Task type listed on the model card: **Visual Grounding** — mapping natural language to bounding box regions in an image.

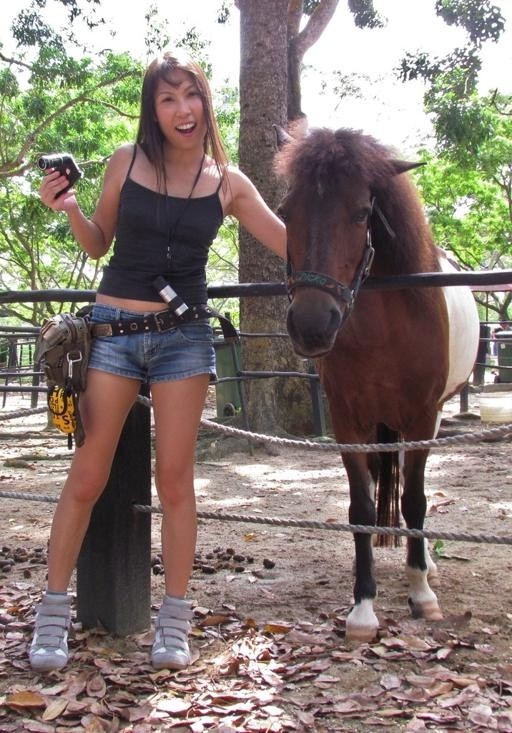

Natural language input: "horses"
[273,122,480,644]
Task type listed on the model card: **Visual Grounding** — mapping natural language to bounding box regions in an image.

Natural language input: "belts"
[93,304,240,347]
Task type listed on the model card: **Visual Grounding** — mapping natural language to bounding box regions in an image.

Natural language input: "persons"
[28,50,289,672]
[500,319,512,331]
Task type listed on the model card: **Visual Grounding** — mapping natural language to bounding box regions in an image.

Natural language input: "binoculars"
[149,274,190,318]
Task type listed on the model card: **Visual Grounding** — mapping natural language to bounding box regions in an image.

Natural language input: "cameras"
[38,150,84,198]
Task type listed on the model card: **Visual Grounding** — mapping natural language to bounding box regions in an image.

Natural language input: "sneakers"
[27,594,69,676]
[150,594,195,674]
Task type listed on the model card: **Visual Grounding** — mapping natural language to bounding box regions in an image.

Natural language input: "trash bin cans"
[495,330,512,383]
[213,335,242,417]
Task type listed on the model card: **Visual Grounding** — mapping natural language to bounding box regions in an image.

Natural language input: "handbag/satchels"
[30,309,92,449]
